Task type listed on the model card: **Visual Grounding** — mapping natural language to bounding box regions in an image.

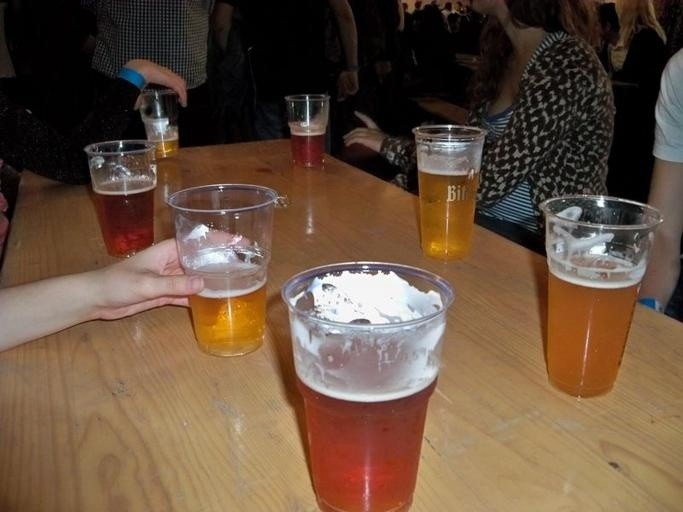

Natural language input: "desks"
[1,135,680,512]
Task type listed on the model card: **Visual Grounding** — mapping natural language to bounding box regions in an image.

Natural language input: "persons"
[630,46,683,311]
[0,228,268,357]
[0,1,682,264]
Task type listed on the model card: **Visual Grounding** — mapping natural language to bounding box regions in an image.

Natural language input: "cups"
[282,92,333,170]
[136,89,182,160]
[82,138,157,257]
[164,181,277,358]
[536,192,664,400]
[281,260,453,512]
[412,124,490,259]
